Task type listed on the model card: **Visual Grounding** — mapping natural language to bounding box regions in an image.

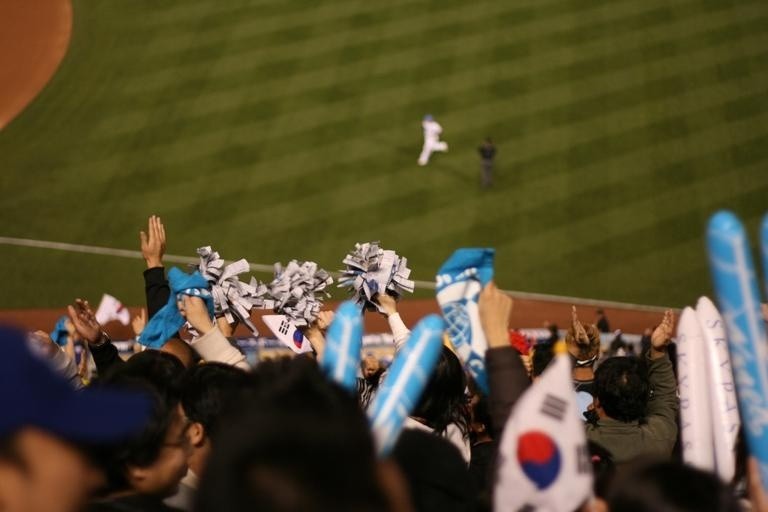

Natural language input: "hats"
[566,322,600,360]
[0,324,151,448]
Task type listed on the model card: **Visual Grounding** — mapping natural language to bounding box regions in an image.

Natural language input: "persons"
[417,116,448,166]
[478,135,498,185]
